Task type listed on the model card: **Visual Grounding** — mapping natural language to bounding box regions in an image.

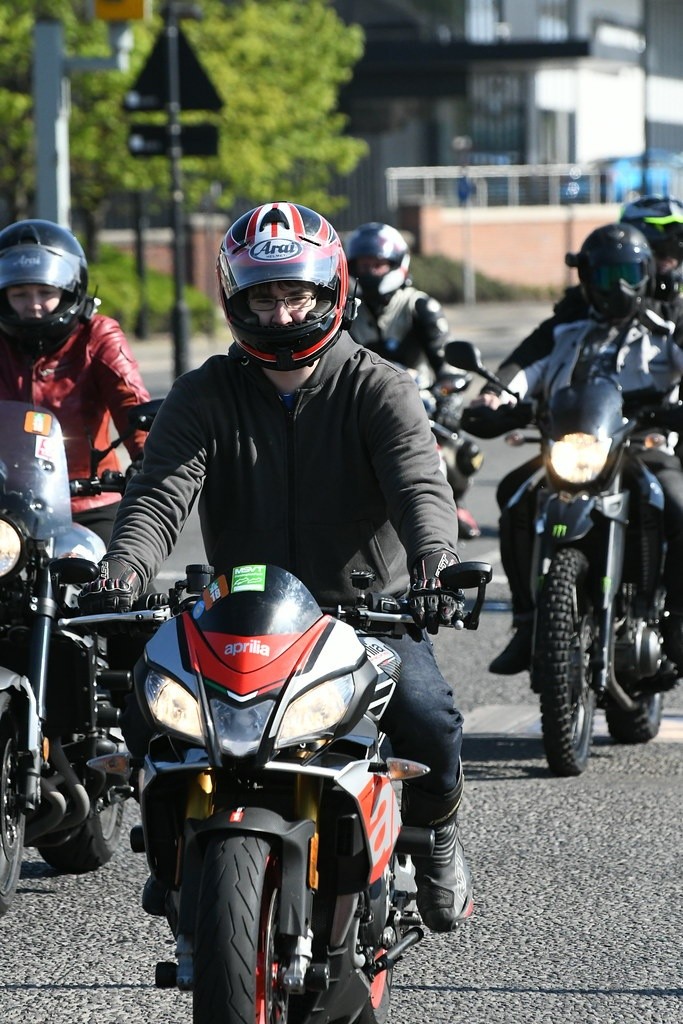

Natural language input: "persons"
[0,220,162,806]
[81,185,475,987]
[461,195,683,676]
[343,220,485,541]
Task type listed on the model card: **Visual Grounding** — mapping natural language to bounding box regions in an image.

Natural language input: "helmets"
[215,202,350,369]
[621,196,683,295]
[578,223,655,321]
[344,222,412,298]
[0,217,88,348]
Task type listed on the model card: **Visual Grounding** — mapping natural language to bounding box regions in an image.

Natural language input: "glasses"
[246,294,319,312]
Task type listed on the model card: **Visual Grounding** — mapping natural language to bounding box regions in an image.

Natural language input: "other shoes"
[416,828,474,932]
[489,633,529,676]
[139,877,164,916]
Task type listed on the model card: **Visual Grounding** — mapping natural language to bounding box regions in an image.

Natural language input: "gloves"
[76,559,148,635]
[404,549,463,644]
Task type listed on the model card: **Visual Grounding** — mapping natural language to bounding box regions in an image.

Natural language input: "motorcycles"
[0,276,681,1024]
